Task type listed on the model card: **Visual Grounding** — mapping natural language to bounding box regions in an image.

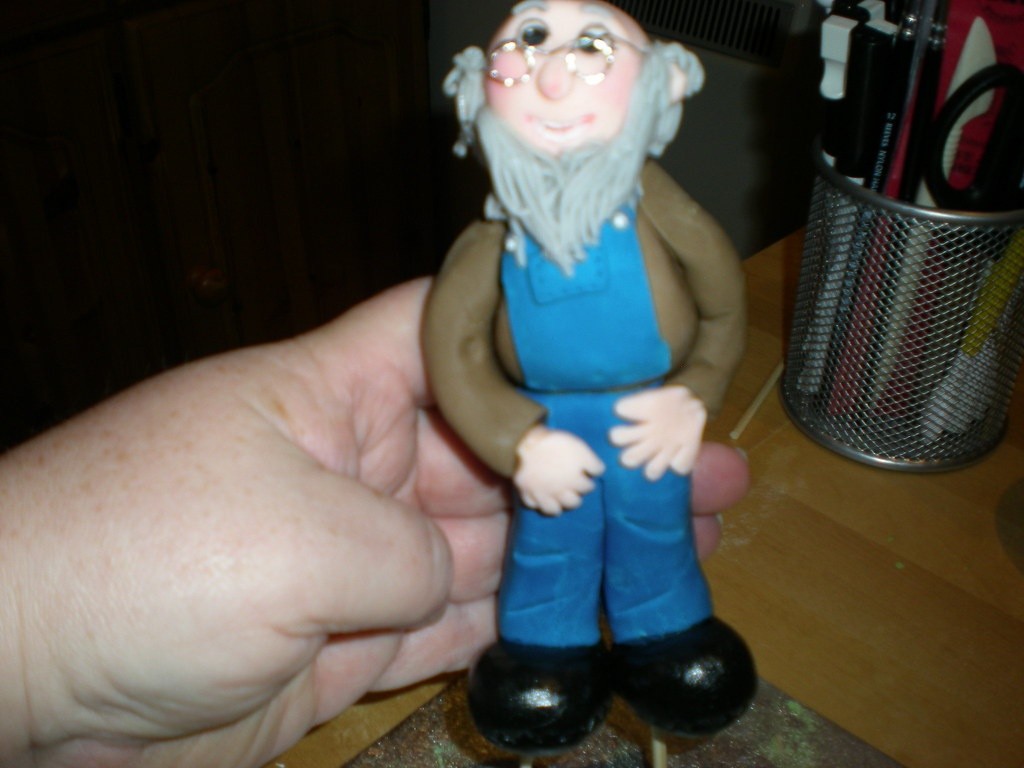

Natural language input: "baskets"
[782,141,1023,471]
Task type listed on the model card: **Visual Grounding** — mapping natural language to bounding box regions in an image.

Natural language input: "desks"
[245,227,1024,768]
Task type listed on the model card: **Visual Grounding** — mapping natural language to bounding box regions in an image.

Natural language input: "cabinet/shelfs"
[0,0,445,455]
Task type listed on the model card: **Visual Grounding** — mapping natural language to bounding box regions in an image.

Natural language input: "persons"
[421,0,759,754]
[0,270,750,767]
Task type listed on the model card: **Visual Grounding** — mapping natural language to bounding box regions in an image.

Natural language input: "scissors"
[909,67,1024,414]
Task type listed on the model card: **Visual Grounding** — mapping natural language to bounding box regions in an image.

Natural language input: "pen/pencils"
[799,0,944,414]
[919,224,1024,441]
